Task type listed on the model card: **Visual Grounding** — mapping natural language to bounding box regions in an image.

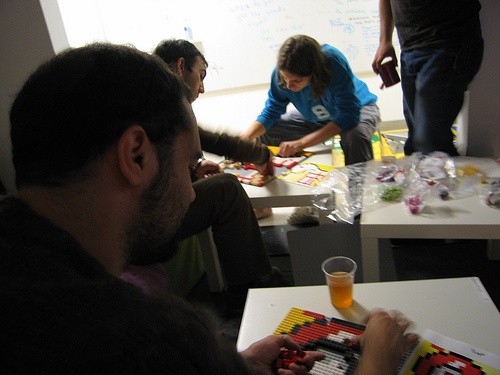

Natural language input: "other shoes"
[254,206,272,221]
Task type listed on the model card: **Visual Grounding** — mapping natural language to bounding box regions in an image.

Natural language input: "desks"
[217,145,337,224]
[359,158,500,283]
[236,276,500,375]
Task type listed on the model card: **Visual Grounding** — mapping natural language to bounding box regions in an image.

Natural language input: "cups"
[321,256,358,311]
[379,60,400,87]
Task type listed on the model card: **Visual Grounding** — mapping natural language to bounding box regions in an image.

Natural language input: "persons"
[151,39,280,293]
[372,0,484,248]
[237,35,382,224]
[0,44,418,375]
